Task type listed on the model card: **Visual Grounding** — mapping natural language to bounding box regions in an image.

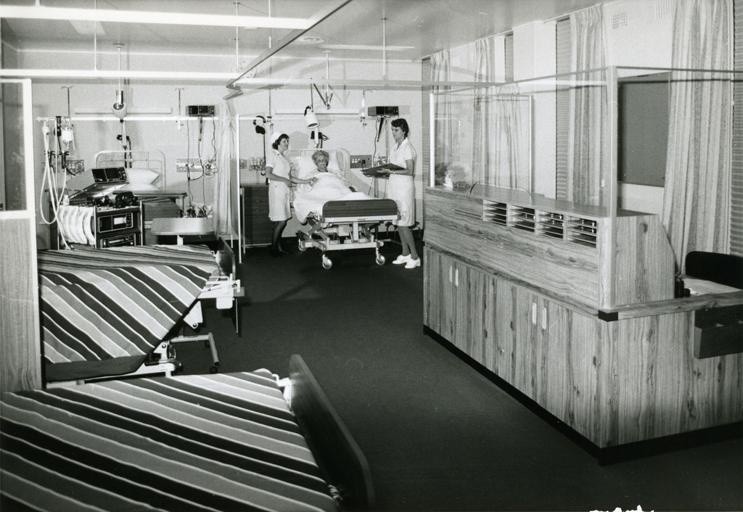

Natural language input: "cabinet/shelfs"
[422,245,608,449]
[238,183,278,248]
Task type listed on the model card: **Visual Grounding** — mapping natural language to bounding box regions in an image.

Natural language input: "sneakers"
[389,252,421,271]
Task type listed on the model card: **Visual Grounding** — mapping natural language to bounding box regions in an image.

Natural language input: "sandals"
[269,243,289,258]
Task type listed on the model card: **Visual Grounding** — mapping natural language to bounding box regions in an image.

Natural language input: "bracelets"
[391,169,395,175]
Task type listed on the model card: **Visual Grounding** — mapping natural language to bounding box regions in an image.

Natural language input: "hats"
[270,131,282,145]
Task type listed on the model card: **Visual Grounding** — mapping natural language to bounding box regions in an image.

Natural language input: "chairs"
[684,251,742,325]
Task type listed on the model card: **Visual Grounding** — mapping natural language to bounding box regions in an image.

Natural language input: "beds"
[285,147,400,271]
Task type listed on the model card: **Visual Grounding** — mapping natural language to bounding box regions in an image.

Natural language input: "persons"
[377,118,421,269]
[303,150,355,191]
[263,128,318,259]
